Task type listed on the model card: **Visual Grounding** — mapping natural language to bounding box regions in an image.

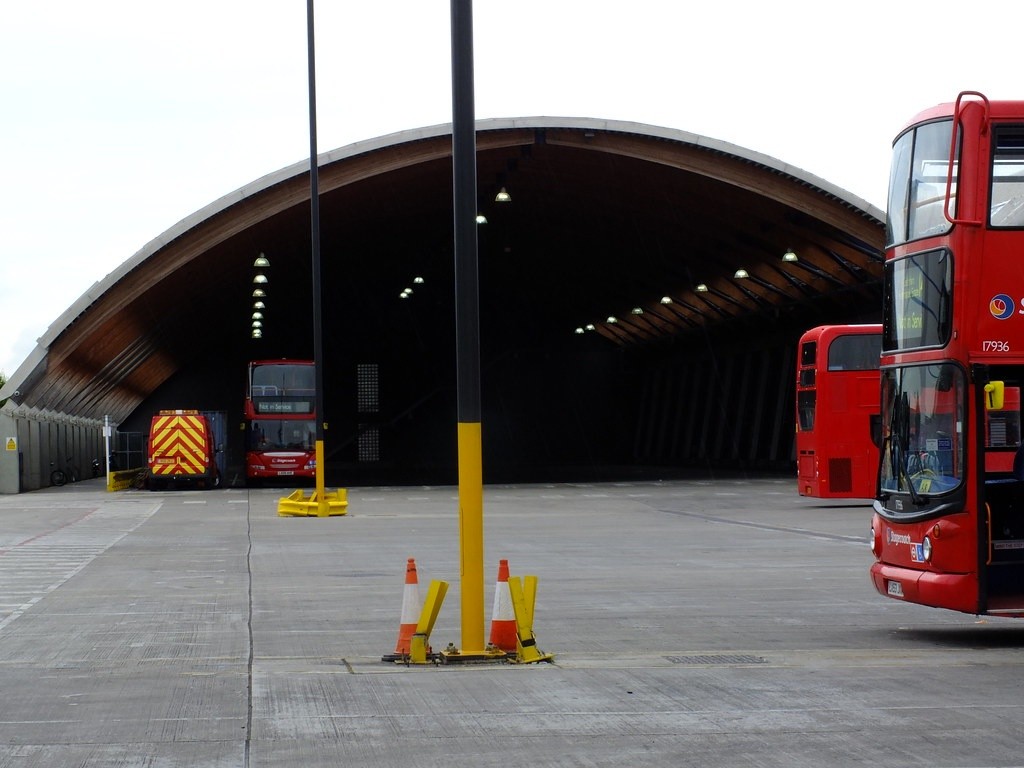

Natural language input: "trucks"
[146,410,228,487]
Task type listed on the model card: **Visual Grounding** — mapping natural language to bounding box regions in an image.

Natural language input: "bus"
[795,324,884,499]
[243,359,316,487]
[870,91,1024,620]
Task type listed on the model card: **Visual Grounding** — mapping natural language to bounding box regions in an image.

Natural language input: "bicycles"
[50,457,78,487]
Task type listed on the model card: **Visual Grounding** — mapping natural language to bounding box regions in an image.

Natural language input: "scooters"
[90,458,102,479]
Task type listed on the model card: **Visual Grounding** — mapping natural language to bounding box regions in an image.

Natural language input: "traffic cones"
[382,557,438,662]
[486,559,520,662]
